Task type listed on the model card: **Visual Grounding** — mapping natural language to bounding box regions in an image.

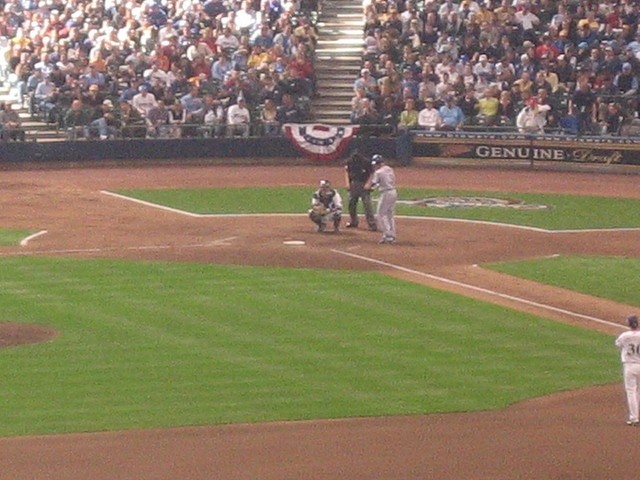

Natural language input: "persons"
[364,154,397,244]
[615,316,640,426]
[308,180,343,236]
[344,147,377,230]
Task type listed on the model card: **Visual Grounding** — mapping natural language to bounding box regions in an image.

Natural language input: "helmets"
[371,154,383,166]
